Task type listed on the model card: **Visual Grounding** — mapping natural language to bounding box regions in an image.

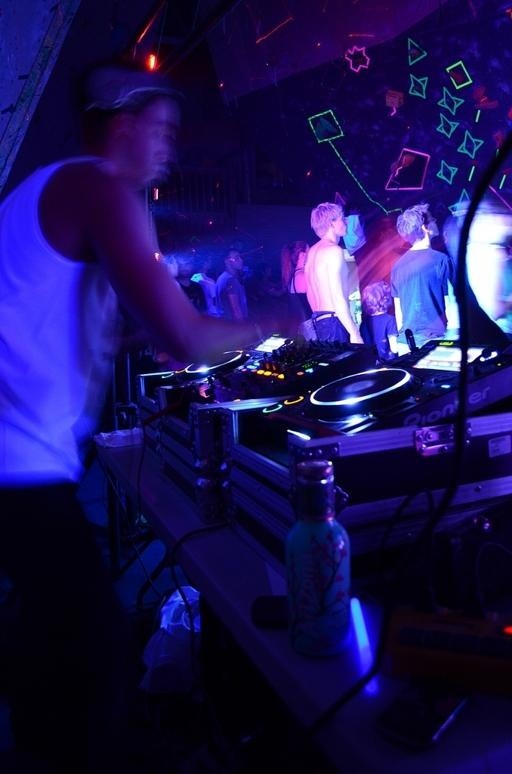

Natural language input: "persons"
[304,203,365,345]
[0,65,312,774]
[487,233,512,334]
[363,284,400,361]
[166,242,315,342]
[390,204,451,354]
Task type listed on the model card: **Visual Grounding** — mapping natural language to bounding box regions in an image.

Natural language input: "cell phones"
[372,679,474,752]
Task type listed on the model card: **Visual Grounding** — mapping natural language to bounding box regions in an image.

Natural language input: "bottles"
[286,460,349,656]
